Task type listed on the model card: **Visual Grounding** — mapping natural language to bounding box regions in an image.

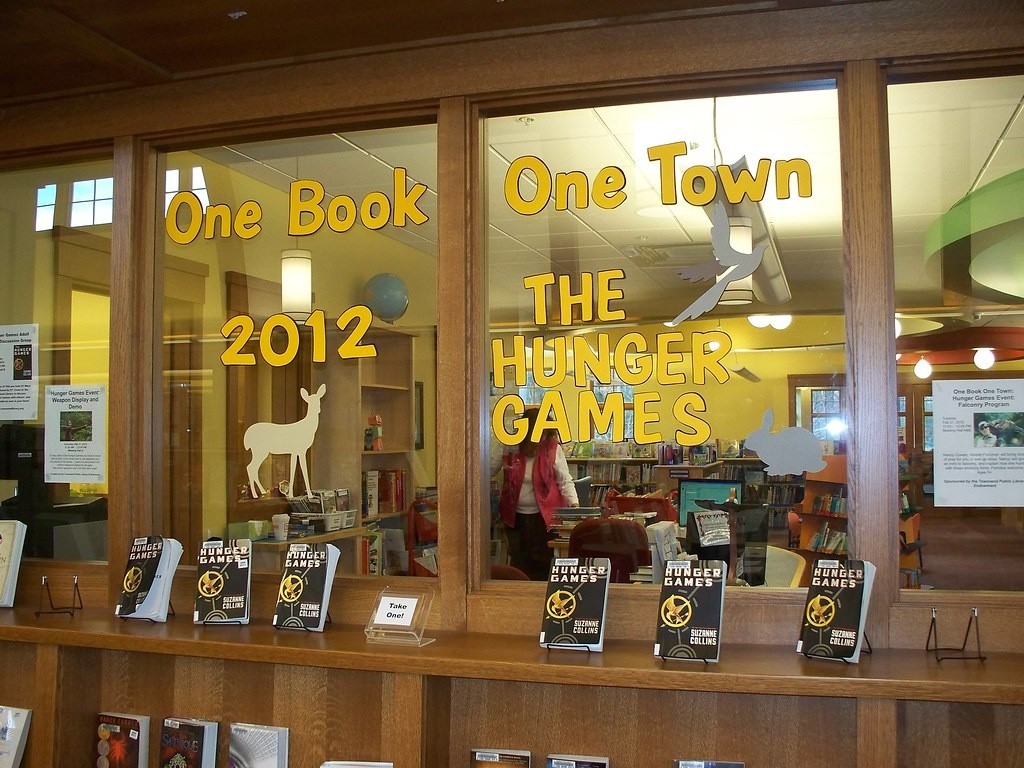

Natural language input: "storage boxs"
[302,509,357,532]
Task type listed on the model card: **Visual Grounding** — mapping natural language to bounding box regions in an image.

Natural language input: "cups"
[272,514,290,541]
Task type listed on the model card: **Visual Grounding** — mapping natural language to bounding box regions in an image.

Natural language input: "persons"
[976,421,996,448]
[490,408,579,581]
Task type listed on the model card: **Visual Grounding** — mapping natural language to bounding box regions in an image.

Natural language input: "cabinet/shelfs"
[310,316,415,527]
[566,456,849,589]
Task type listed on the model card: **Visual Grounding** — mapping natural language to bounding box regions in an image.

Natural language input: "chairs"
[566,519,652,585]
[752,546,806,589]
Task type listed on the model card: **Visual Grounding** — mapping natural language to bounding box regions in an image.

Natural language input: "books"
[0,706,33,768]
[470,749,531,768]
[549,440,804,581]
[546,754,610,768]
[228,723,289,768]
[539,557,611,652]
[0,520,27,608]
[268,468,407,575]
[673,759,745,768]
[796,559,877,664]
[115,536,184,622]
[193,539,252,625]
[272,543,340,632]
[808,492,909,551]
[159,717,218,768]
[320,760,393,768]
[94,712,150,768]
[653,560,727,663]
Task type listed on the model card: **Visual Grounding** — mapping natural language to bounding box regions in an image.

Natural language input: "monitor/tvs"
[678,477,744,527]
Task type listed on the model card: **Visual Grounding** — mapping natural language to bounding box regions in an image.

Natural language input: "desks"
[0,603,1024,768]
[251,526,367,577]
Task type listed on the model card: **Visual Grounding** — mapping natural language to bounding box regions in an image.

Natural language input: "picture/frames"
[415,381,424,450]
